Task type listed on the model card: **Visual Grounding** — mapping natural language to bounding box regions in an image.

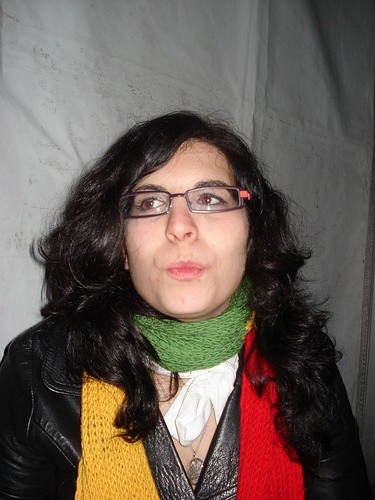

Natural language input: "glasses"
[115,187,250,219]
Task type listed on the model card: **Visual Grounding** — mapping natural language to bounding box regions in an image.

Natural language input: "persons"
[0,111,375,500]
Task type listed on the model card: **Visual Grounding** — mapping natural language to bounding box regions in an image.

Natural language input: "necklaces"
[155,375,211,485]
